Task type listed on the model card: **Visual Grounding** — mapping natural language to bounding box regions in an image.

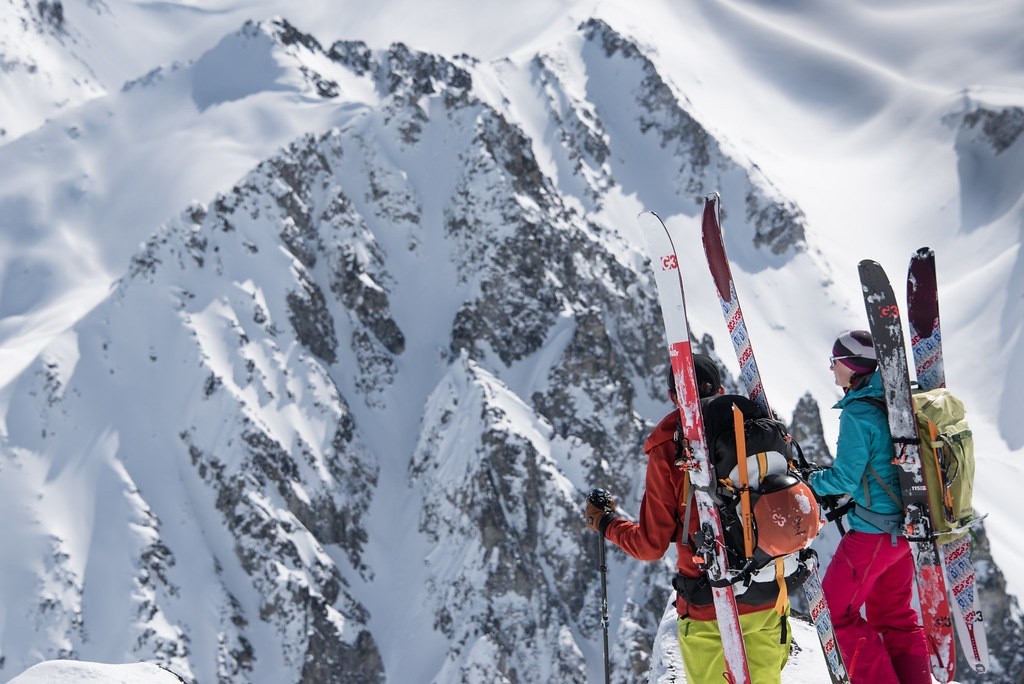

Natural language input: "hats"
[833,330,877,374]
[667,353,722,399]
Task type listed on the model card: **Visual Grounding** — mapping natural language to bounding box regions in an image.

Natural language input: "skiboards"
[634,189,851,683]
[857,245,989,683]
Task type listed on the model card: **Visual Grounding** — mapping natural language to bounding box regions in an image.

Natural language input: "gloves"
[585,493,616,537]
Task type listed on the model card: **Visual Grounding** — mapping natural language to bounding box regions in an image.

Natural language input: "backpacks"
[855,388,977,546]
[673,391,823,606]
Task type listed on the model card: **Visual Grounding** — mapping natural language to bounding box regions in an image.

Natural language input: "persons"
[584,352,793,684]
[789,331,933,684]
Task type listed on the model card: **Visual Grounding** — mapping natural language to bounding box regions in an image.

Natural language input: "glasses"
[829,354,863,366]
